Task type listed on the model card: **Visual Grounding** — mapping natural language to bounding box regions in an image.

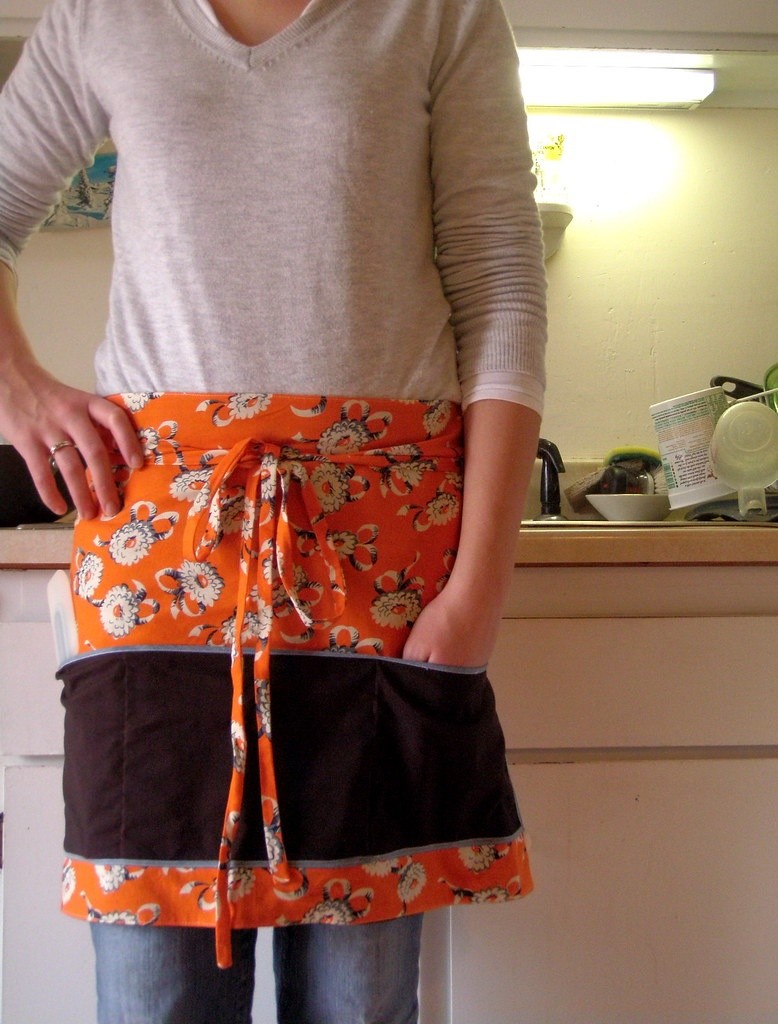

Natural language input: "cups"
[649,386,737,510]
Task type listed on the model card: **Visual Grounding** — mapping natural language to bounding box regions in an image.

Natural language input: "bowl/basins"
[585,494,672,521]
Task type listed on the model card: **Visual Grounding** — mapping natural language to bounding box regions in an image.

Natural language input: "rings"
[50,441,72,453]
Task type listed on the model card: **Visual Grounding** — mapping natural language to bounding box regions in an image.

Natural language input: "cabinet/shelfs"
[0,529,778,1024]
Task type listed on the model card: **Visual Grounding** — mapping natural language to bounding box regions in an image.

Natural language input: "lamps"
[518,62,714,112]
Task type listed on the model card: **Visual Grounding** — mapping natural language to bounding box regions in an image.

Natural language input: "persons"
[0,0,547,1024]
